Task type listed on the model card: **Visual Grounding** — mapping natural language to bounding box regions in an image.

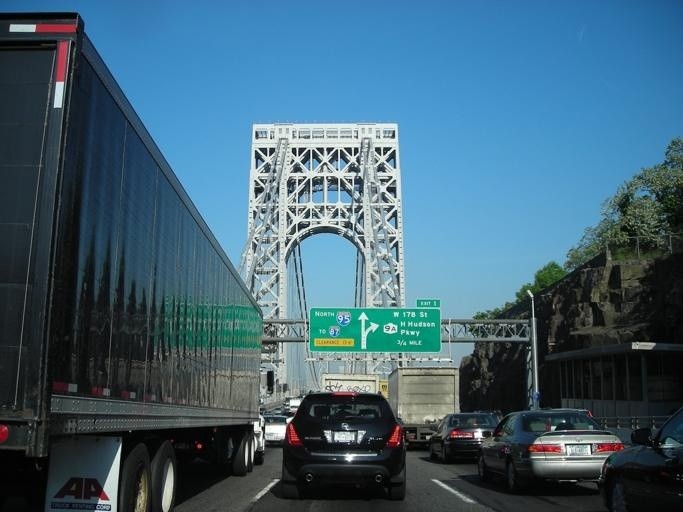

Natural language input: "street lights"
[526,287,540,405]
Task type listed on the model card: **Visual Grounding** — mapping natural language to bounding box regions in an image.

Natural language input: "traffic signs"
[309,307,442,353]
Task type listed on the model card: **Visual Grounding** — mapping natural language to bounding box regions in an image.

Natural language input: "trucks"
[319,372,380,395]
[0,7,275,512]
[387,365,463,451]
